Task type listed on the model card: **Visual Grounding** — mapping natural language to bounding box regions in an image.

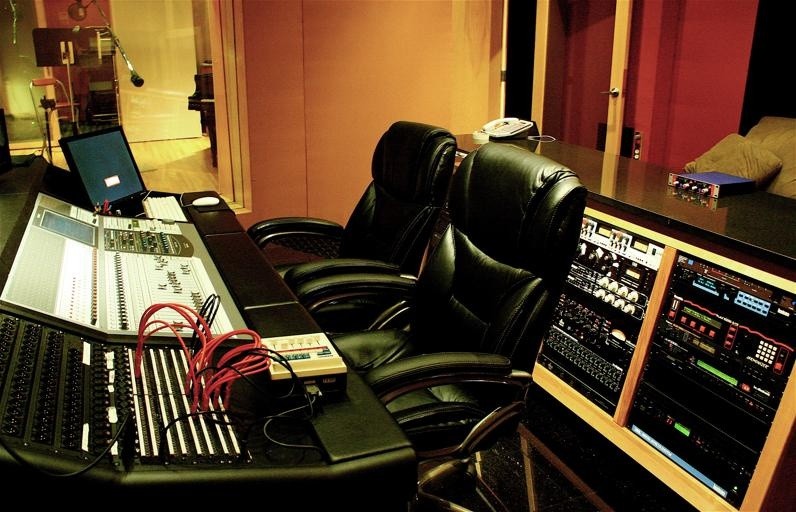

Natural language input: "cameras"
[41,99,55,110]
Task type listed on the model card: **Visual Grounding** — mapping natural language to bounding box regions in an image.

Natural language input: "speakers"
[0,109,13,176]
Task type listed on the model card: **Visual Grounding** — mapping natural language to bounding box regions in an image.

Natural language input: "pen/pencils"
[94,199,112,216]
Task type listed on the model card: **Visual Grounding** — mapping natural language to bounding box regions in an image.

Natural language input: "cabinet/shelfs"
[79,30,122,133]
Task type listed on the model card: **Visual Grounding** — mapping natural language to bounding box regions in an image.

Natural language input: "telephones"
[480,118,540,140]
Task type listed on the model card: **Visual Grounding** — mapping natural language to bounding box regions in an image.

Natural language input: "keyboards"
[141,196,189,222]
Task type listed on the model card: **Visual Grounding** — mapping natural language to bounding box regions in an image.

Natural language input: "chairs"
[28,77,79,166]
[294,142,590,512]
[245,119,459,334]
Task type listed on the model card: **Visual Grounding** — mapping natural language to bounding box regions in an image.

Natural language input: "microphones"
[76,0,85,18]
[23,154,35,167]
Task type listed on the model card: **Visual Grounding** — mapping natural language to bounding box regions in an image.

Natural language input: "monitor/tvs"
[59,125,147,215]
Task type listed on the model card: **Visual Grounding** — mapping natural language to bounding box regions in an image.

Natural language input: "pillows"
[687,132,782,187]
[733,114,796,197]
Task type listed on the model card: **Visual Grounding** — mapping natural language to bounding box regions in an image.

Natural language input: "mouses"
[192,196,220,207]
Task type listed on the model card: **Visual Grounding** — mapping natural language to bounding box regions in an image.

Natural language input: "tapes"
[473,133,489,140]
[472,140,489,144]
